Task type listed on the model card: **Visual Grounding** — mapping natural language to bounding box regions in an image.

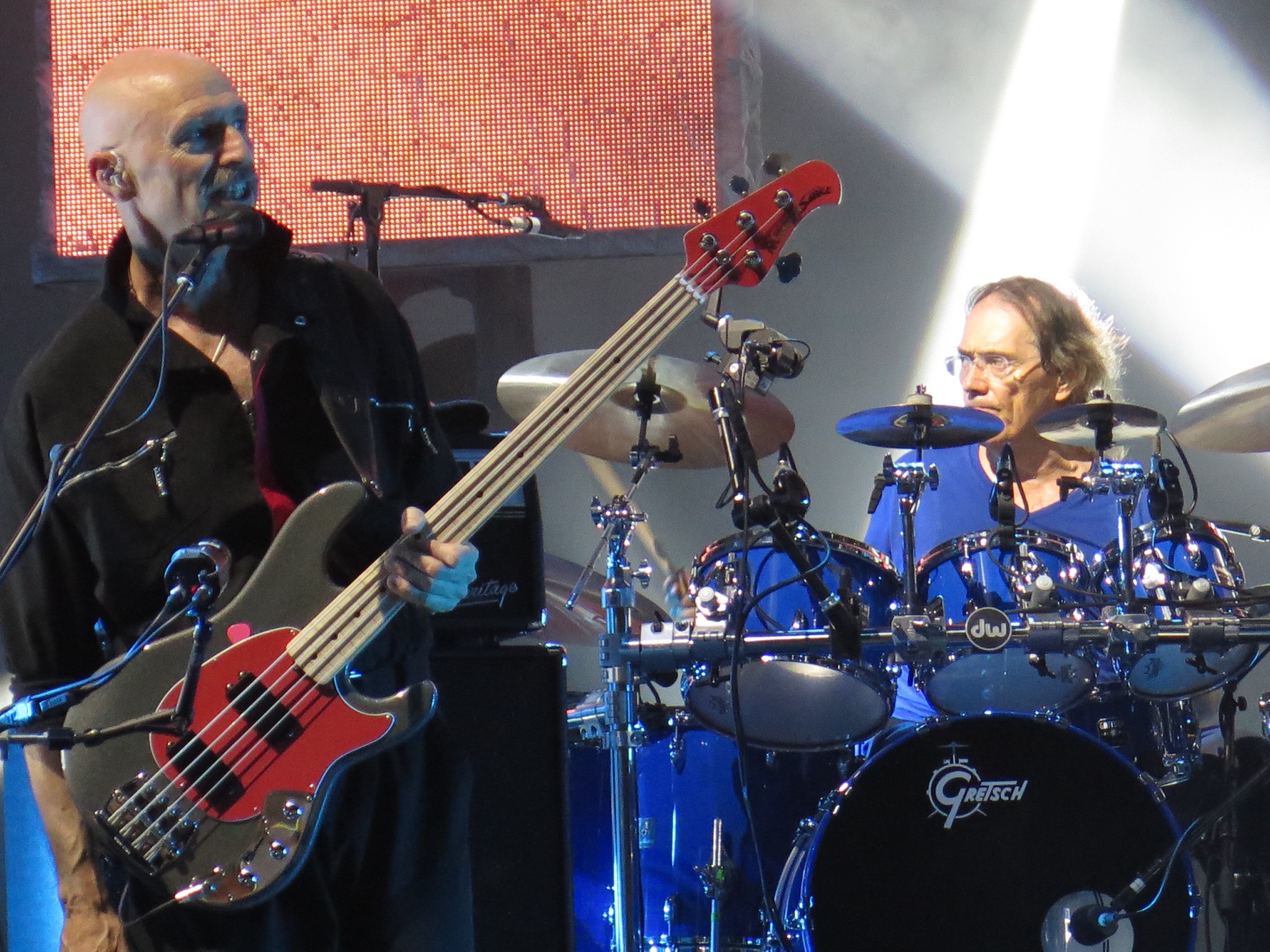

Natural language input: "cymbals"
[1033,399,1167,447]
[496,349,795,470]
[835,405,1005,449]
[1171,362,1270,453]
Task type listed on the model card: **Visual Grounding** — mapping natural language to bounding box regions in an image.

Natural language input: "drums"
[570,729,749,952]
[915,528,1099,716]
[1091,516,1259,702]
[680,528,901,753]
[766,710,1202,952]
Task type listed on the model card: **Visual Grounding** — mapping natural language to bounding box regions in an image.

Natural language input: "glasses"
[945,353,1044,378]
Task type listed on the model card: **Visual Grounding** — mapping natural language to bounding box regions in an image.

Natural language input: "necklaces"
[123,264,260,370]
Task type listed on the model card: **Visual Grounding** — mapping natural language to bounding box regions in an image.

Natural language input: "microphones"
[169,205,265,251]
[498,214,585,240]
[744,326,805,384]
[1145,434,1171,522]
[770,447,797,555]
[988,445,1016,523]
[163,540,231,618]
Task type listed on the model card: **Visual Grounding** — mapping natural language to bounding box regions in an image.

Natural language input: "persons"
[1,44,478,950]
[731,278,1177,924]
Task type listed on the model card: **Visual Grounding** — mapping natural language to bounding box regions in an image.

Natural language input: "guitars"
[60,153,842,910]
